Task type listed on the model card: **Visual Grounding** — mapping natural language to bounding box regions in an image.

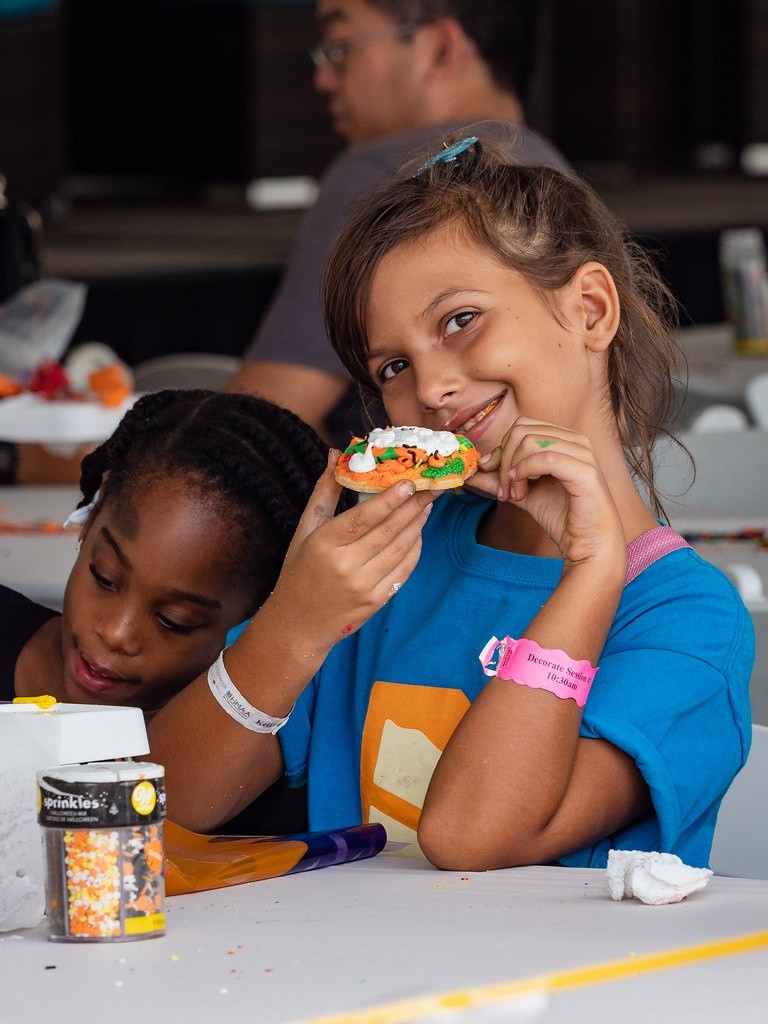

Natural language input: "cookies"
[334,433,481,493]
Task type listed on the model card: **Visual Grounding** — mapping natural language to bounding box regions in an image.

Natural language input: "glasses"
[392,136,483,185]
[313,24,456,73]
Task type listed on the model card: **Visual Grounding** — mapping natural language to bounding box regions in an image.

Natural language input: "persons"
[230,0,590,425]
[149,134,756,875]
[0,390,360,735]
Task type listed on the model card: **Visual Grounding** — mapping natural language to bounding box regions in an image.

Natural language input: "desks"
[46,175,768,283]
[0,856,768,1024]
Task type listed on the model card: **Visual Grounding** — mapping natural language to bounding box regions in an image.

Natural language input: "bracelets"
[479,631,601,707]
[207,644,297,733]
